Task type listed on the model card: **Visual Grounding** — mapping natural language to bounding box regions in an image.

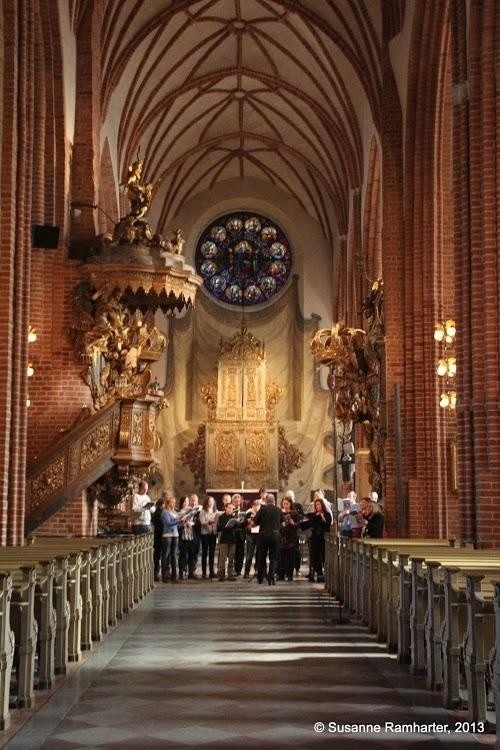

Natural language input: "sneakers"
[154,570,324,585]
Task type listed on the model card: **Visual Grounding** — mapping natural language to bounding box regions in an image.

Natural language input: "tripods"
[248,534,272,583]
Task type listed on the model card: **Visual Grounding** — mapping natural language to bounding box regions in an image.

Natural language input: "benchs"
[0,525,156,734]
[322,527,500,736]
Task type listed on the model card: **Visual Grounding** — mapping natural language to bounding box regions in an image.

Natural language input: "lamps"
[436,356,457,379]
[439,388,457,411]
[433,318,458,347]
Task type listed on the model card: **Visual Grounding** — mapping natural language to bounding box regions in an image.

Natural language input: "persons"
[126,159,148,229]
[132,478,385,585]
